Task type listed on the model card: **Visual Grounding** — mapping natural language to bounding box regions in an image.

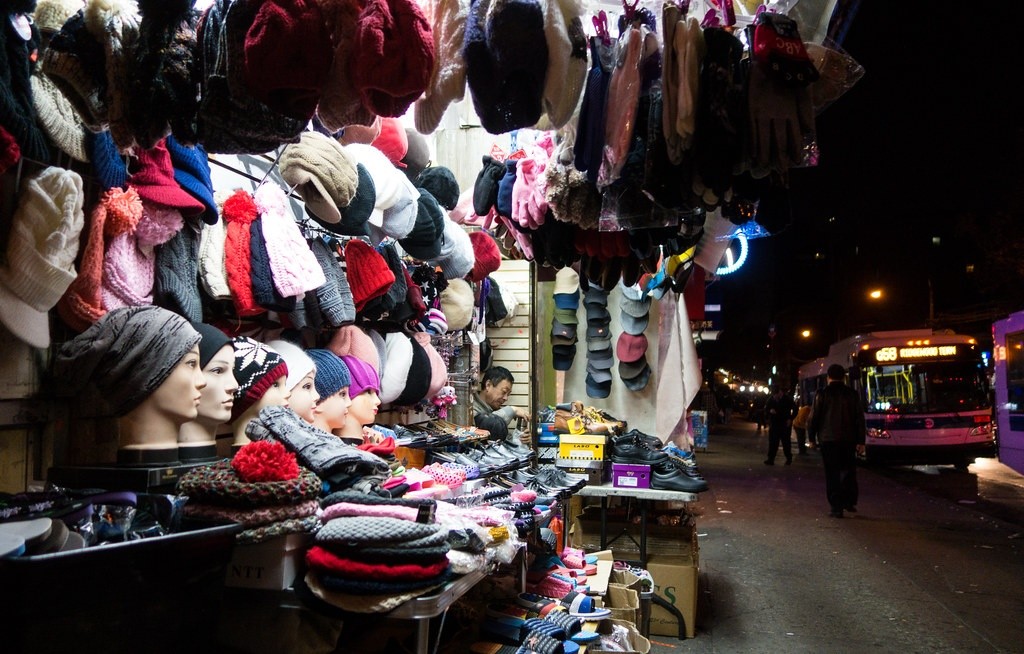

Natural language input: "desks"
[577,482,697,641]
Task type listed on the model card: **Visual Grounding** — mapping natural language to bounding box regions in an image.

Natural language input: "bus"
[797,328,1000,472]
[991,311,1024,475]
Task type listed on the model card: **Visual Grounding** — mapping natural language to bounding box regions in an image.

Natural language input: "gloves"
[470,5,864,293]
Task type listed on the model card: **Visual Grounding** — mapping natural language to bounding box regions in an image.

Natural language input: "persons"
[807,364,866,518]
[266,339,320,422]
[178,322,238,446]
[473,366,531,444]
[230,335,291,446]
[56,306,208,448]
[755,392,766,431]
[333,354,382,439]
[763,386,799,465]
[794,405,811,456]
[303,349,352,434]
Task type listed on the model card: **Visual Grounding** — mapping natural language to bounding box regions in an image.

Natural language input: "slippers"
[492,543,654,654]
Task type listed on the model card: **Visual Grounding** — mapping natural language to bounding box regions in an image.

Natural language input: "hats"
[550,262,693,398]
[175,408,538,613]
[0,0,570,426]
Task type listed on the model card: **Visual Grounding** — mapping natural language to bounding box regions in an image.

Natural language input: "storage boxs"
[569,506,699,654]
[389,445,423,470]
[537,419,611,485]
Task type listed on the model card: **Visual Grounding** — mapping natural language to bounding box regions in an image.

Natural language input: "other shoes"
[763,459,774,466]
[842,502,857,513]
[799,452,810,457]
[785,457,792,465]
[828,512,844,519]
[362,416,586,534]
[536,399,710,492]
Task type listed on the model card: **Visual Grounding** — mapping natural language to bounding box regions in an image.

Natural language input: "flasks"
[517,418,529,435]
